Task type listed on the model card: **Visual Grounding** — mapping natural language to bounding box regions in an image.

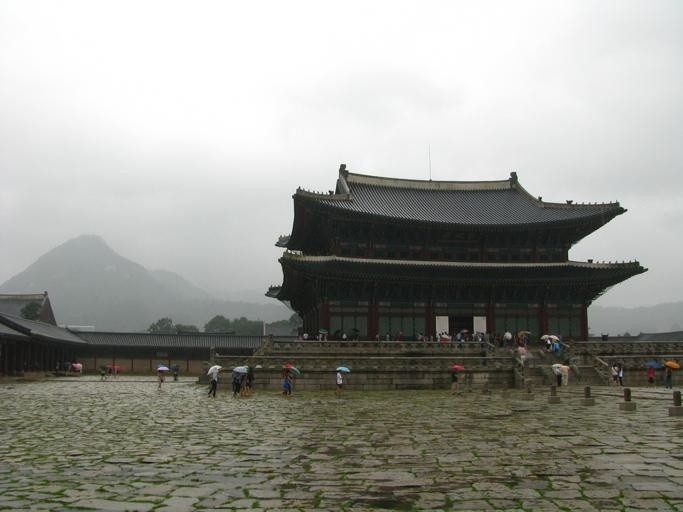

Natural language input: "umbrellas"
[551,363,562,368]
[282,364,300,376]
[154,366,169,372]
[664,360,680,369]
[644,359,664,370]
[450,363,464,372]
[335,366,351,375]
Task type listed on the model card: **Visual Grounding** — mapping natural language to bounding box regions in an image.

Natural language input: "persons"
[611,365,618,384]
[618,367,623,386]
[334,370,342,397]
[555,367,561,386]
[205,364,255,398]
[281,370,294,396]
[560,362,569,386]
[450,368,460,395]
[663,365,673,390]
[157,370,166,391]
[173,365,180,380]
[647,367,656,387]
[54,358,122,380]
[299,325,561,362]
